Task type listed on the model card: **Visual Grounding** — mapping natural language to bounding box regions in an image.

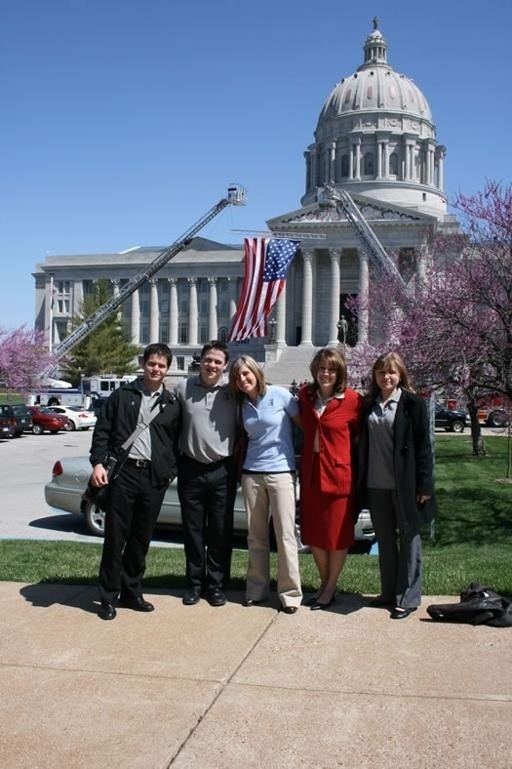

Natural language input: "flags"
[226,236,301,342]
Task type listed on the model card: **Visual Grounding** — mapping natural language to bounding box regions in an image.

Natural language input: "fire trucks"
[314,179,512,427]
[26,182,250,409]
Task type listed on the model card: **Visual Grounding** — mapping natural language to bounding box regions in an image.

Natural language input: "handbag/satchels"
[85,451,119,512]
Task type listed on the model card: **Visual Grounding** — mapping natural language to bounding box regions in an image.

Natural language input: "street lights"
[268,319,278,346]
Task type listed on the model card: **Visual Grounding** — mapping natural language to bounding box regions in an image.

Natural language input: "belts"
[126,458,151,469]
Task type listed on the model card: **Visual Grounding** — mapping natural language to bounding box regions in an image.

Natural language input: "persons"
[87,341,183,622]
[362,354,433,622]
[173,340,249,610]
[298,348,365,610]
[229,353,303,613]
[335,313,349,343]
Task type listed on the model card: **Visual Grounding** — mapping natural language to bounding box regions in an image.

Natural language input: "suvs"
[0,403,18,441]
[9,404,33,439]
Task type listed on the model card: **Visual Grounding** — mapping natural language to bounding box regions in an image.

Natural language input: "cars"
[36,406,98,432]
[434,402,466,433]
[26,406,70,435]
[45,452,377,553]
[86,391,110,419]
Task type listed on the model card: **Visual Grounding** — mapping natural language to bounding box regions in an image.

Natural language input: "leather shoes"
[370,595,396,607]
[391,605,417,618]
[284,607,296,613]
[311,589,337,610]
[184,591,200,604]
[243,599,256,606]
[100,595,116,619]
[120,595,154,611]
[303,595,317,605]
[201,587,227,606]
